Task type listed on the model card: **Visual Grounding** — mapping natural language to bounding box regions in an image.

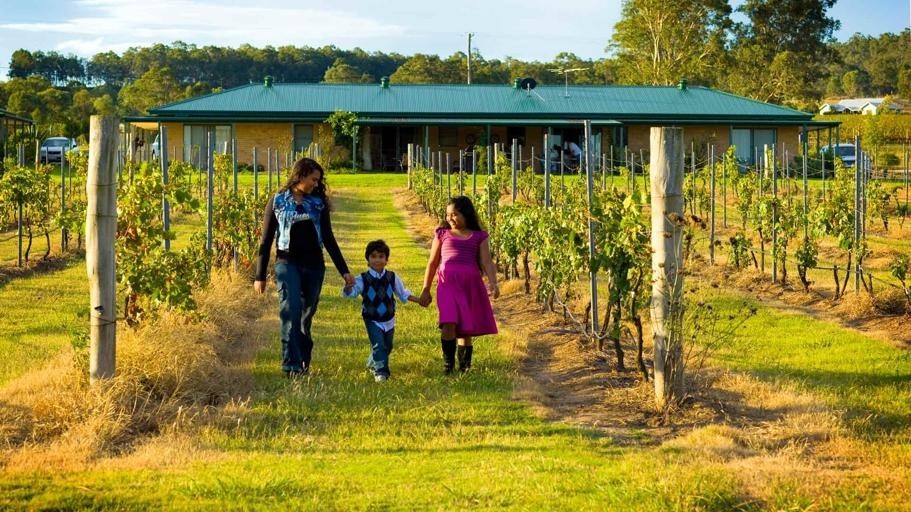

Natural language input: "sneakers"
[286,360,311,379]
[369,368,388,384]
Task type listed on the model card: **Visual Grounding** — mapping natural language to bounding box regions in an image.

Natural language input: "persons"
[254,157,354,381]
[568,138,582,164]
[420,196,499,375]
[343,240,428,385]
[126,136,144,162]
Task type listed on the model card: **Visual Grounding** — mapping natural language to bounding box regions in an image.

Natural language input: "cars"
[819,143,871,179]
[39,137,79,162]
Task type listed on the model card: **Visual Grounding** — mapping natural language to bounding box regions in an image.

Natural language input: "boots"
[457,344,474,373]
[440,336,457,375]
